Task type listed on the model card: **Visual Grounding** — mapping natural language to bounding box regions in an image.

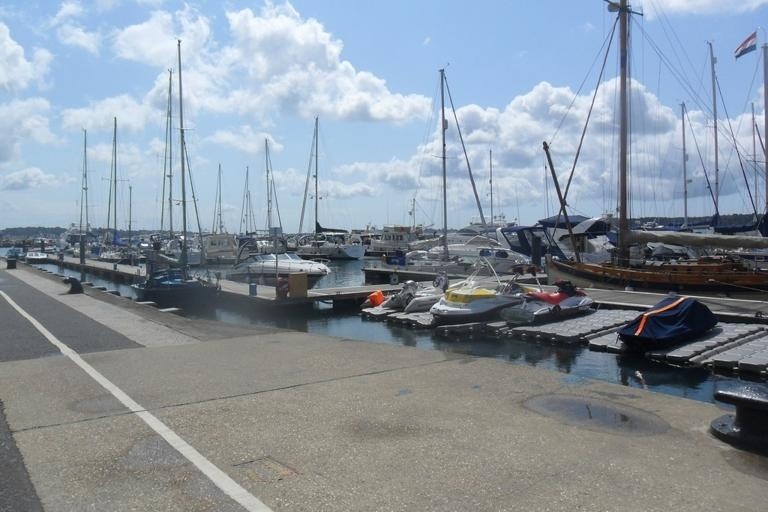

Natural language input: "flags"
[735,31,757,59]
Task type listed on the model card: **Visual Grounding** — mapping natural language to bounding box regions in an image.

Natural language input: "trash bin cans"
[289,272,308,297]
